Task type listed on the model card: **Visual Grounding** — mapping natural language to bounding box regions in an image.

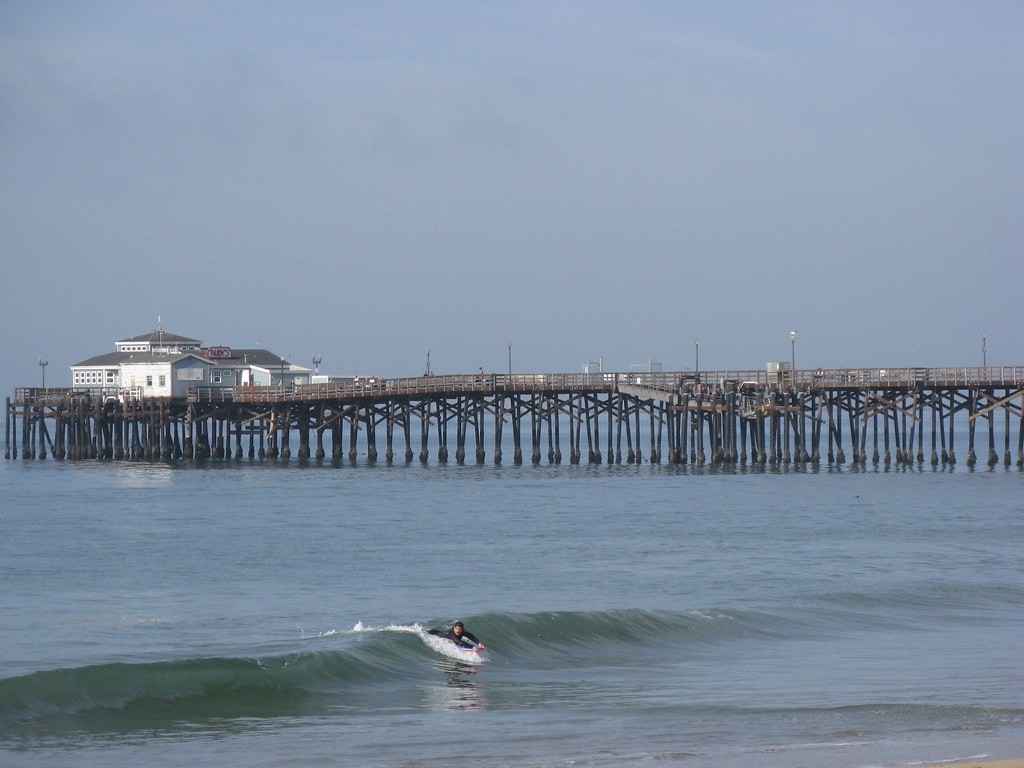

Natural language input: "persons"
[475,367,483,375]
[353,375,377,388]
[816,366,823,382]
[427,622,486,651]
[423,371,435,376]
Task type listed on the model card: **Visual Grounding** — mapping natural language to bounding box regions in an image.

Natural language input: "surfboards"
[418,630,483,652]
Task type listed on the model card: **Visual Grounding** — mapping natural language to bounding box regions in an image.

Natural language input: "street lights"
[427,347,430,376]
[791,330,798,386]
[312,354,323,375]
[508,341,512,381]
[981,332,987,379]
[281,356,284,385]
[38,356,48,388]
[695,338,700,370]
